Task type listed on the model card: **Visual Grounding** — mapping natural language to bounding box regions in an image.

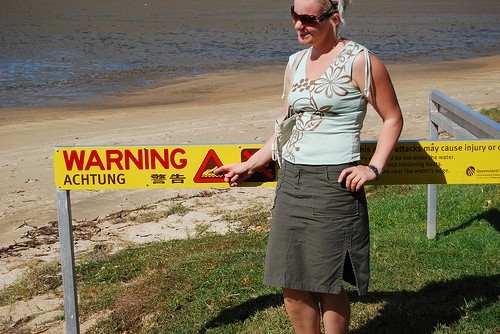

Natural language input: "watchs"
[367,165,380,182]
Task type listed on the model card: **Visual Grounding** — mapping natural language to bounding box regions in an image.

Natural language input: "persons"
[213,0,404,334]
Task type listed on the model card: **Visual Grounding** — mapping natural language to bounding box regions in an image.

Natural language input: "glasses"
[291,5,338,27]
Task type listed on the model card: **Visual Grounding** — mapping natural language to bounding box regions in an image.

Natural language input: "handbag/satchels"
[272,48,308,167]
